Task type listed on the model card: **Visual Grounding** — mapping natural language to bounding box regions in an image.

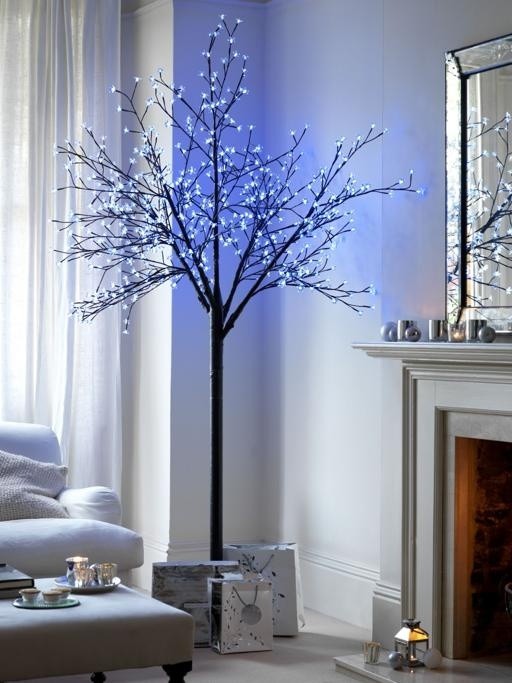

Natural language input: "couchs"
[0,421,144,578]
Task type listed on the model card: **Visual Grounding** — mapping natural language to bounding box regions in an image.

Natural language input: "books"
[0,587,37,600]
[0,563,34,590]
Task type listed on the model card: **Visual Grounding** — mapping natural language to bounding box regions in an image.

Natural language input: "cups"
[74,567,93,588]
[18,588,71,603]
[428,319,449,342]
[397,319,417,341]
[361,641,381,666]
[94,562,116,585]
[446,320,465,341]
[466,318,488,341]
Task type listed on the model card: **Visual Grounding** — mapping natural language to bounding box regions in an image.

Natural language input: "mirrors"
[444,33,512,335]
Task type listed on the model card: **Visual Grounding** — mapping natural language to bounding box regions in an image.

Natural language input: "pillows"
[0,448,72,521]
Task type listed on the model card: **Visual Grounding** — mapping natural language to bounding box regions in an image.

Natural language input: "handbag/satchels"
[224,543,304,637]
[207,577,274,653]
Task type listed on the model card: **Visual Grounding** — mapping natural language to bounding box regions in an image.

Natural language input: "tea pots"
[65,556,94,585]
